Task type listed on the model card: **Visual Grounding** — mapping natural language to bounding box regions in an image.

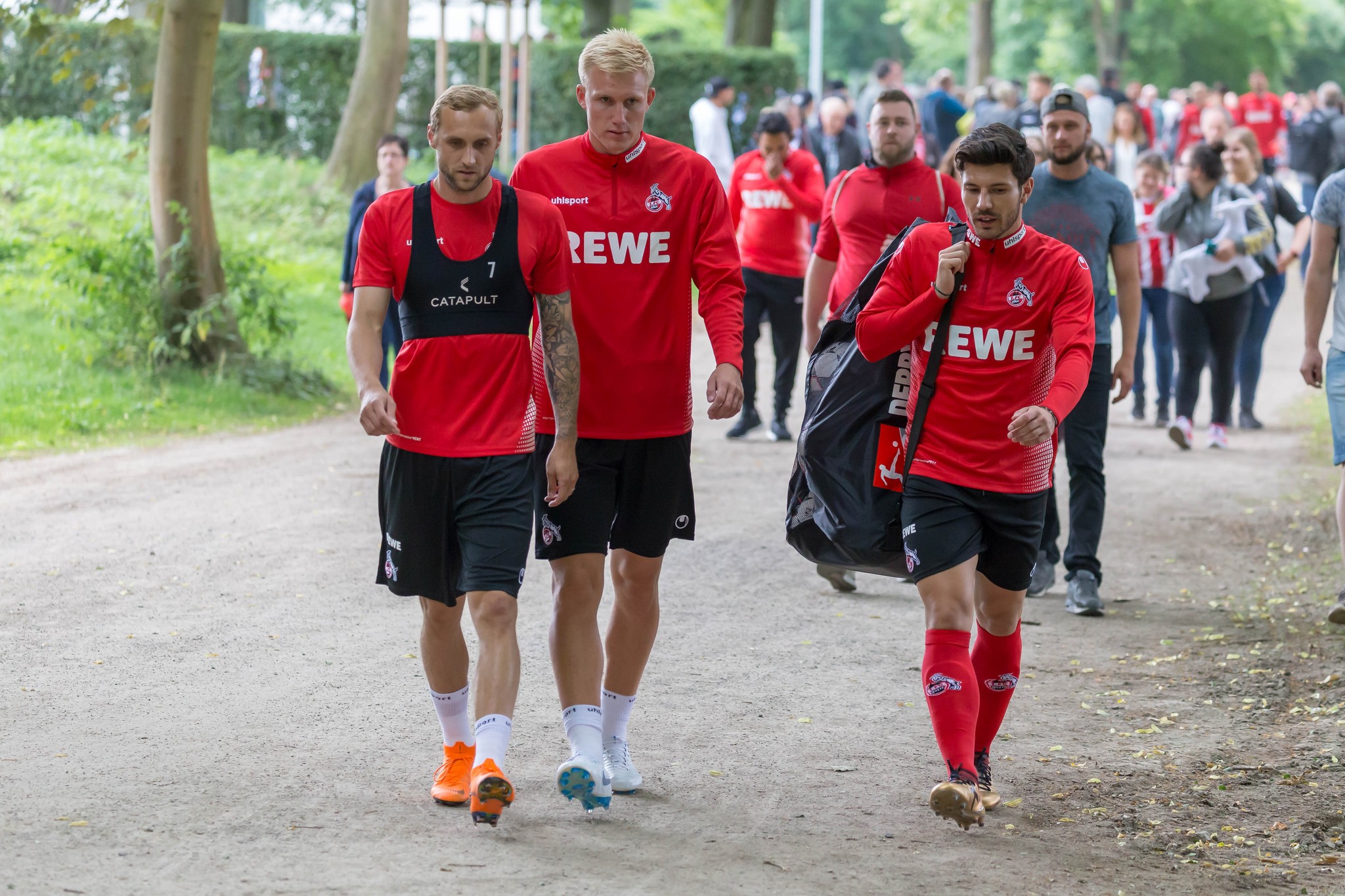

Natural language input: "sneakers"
[1202,422,1228,448]
[929,759,986,831]
[470,758,515,828]
[602,735,642,794]
[431,741,476,807]
[974,747,1001,810]
[1169,416,1195,450]
[556,748,612,815]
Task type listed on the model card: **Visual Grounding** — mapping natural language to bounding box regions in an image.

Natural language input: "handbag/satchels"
[784,206,969,580]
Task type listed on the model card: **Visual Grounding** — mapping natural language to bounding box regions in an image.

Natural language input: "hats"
[1039,88,1089,119]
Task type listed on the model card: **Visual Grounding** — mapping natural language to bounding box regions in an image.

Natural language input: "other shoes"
[1131,397,1146,419]
[1025,550,1056,598]
[770,410,792,441]
[1156,406,1170,427]
[1063,569,1105,616]
[1238,410,1263,429]
[1328,588,1345,624]
[816,564,857,590]
[727,401,764,439]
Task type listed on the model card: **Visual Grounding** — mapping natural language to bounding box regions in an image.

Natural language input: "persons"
[688,77,737,193]
[508,28,745,814]
[776,58,1345,449]
[1021,88,1142,615]
[726,112,826,441]
[1299,170,1345,628]
[801,89,970,594]
[341,135,414,396]
[346,84,582,829]
[855,122,1095,834]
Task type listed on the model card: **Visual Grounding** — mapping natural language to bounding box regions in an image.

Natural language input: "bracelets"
[931,282,951,296]
